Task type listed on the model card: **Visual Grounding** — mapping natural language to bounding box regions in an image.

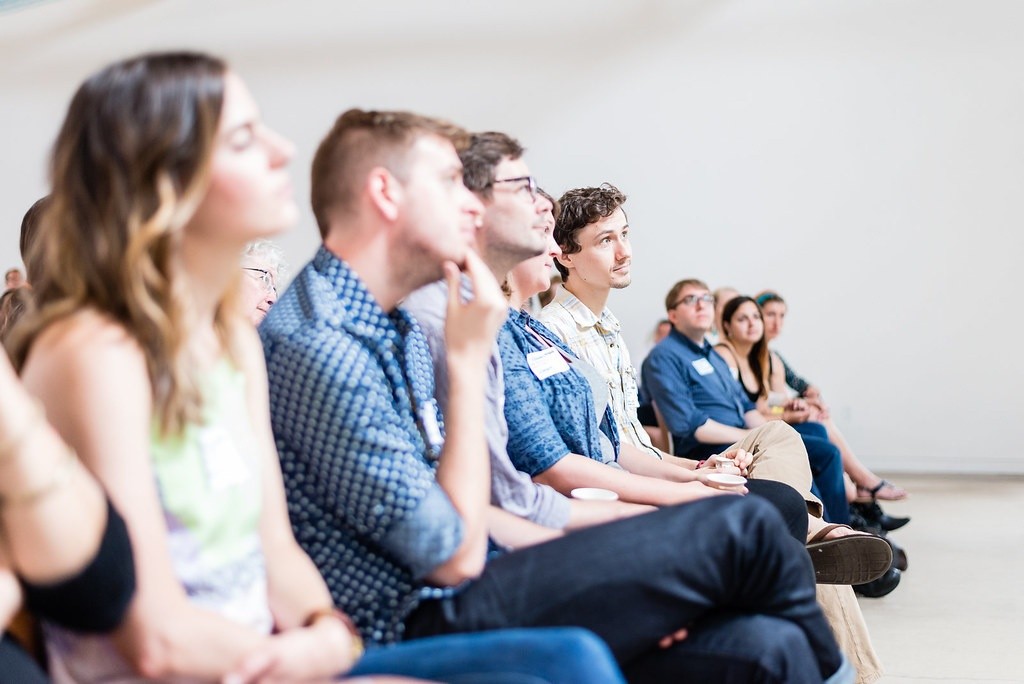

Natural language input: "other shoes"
[879,515,910,531]
[806,524,894,584]
[852,567,901,597]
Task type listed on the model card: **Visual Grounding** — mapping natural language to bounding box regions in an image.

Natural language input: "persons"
[0,52,912,684]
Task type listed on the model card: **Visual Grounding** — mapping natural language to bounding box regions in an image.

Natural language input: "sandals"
[856,480,907,503]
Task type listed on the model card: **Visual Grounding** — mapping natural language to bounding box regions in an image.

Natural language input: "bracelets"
[303,608,363,664]
[0,444,78,511]
[694,460,705,471]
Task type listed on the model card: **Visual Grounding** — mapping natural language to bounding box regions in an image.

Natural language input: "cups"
[569,486,620,503]
[762,390,788,414]
[706,472,748,493]
[713,458,736,468]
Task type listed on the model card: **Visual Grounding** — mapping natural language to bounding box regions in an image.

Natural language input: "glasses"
[495,177,537,200]
[243,268,278,296]
[676,295,714,306]
